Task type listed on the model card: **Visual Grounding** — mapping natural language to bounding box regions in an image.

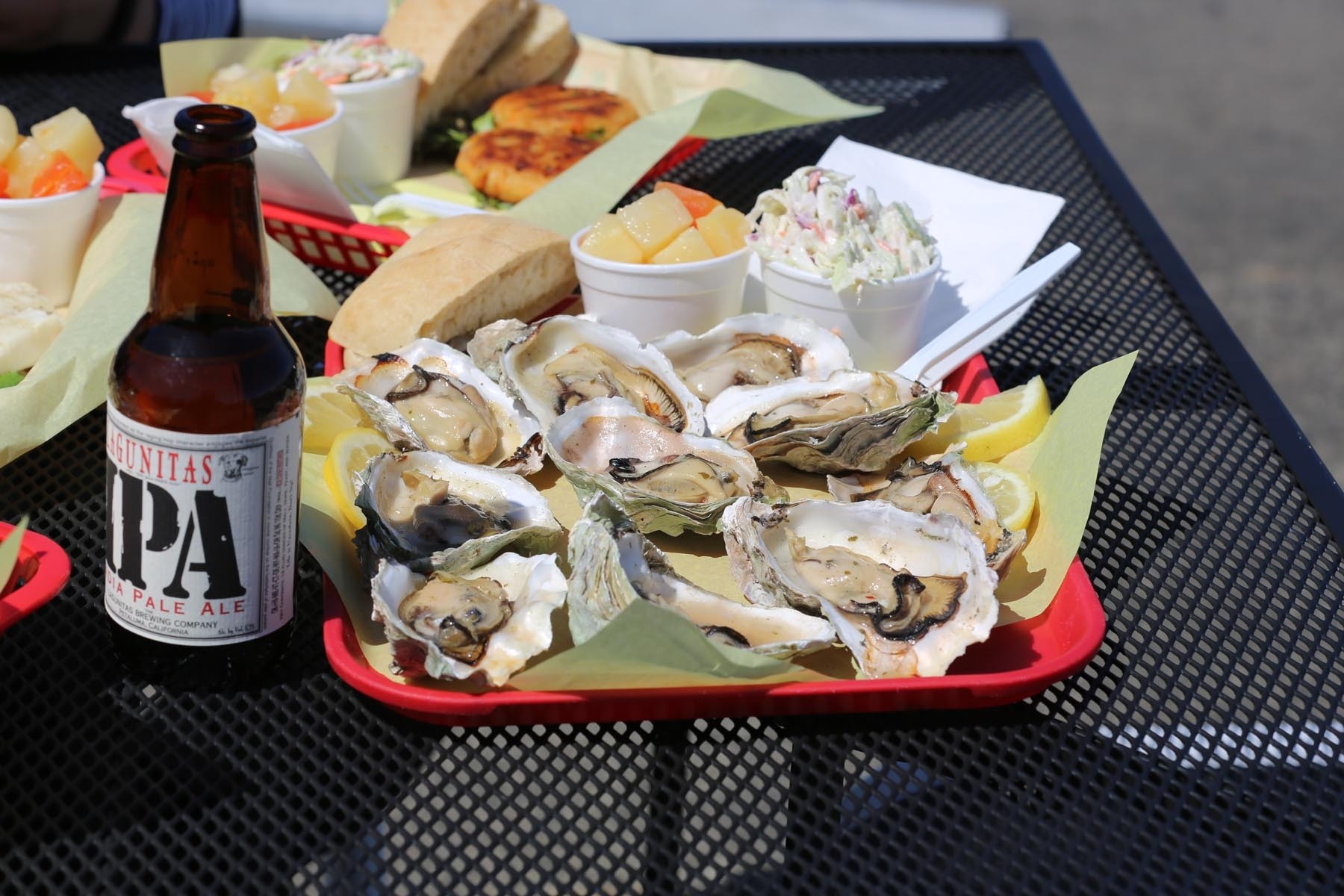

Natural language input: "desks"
[1,37,1344,896]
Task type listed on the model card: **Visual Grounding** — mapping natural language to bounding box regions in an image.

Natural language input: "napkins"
[820,135,1067,356]
[120,97,359,222]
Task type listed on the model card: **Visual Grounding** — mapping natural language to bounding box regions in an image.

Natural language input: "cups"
[279,61,423,188]
[277,96,345,187]
[0,163,103,311]
[571,225,750,338]
[756,237,940,370]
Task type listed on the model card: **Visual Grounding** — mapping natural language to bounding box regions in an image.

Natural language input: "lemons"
[900,376,1050,463]
[964,461,1036,533]
[301,378,368,449]
[324,426,396,530]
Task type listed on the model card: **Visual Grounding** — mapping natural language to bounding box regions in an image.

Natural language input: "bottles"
[101,101,305,672]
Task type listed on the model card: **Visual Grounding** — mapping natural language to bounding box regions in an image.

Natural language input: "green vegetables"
[412,106,510,207]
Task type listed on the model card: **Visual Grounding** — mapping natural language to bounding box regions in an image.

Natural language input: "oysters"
[354,312,1028,691]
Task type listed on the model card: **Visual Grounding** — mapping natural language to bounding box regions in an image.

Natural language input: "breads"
[326,211,574,359]
[377,0,575,140]
[0,280,66,376]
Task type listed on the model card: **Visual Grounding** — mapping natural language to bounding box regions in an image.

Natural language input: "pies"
[454,84,637,205]
